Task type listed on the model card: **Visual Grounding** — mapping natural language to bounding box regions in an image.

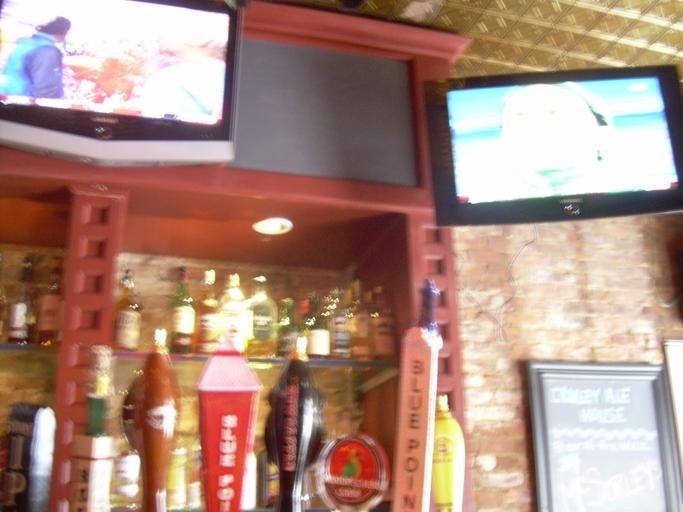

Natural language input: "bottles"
[36,254,64,348]
[113,275,144,349]
[168,264,400,361]
[7,257,39,346]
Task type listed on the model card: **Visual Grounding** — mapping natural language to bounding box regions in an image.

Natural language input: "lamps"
[251,213,293,239]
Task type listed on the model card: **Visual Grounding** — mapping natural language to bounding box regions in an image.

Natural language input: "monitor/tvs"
[421,65,683,227]
[0,0,245,166]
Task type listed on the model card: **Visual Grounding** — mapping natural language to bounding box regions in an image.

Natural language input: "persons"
[1,15,71,98]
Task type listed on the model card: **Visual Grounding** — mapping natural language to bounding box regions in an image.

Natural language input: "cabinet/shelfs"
[1,4,482,512]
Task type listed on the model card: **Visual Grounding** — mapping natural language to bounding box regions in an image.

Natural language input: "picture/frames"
[521,357,683,512]
